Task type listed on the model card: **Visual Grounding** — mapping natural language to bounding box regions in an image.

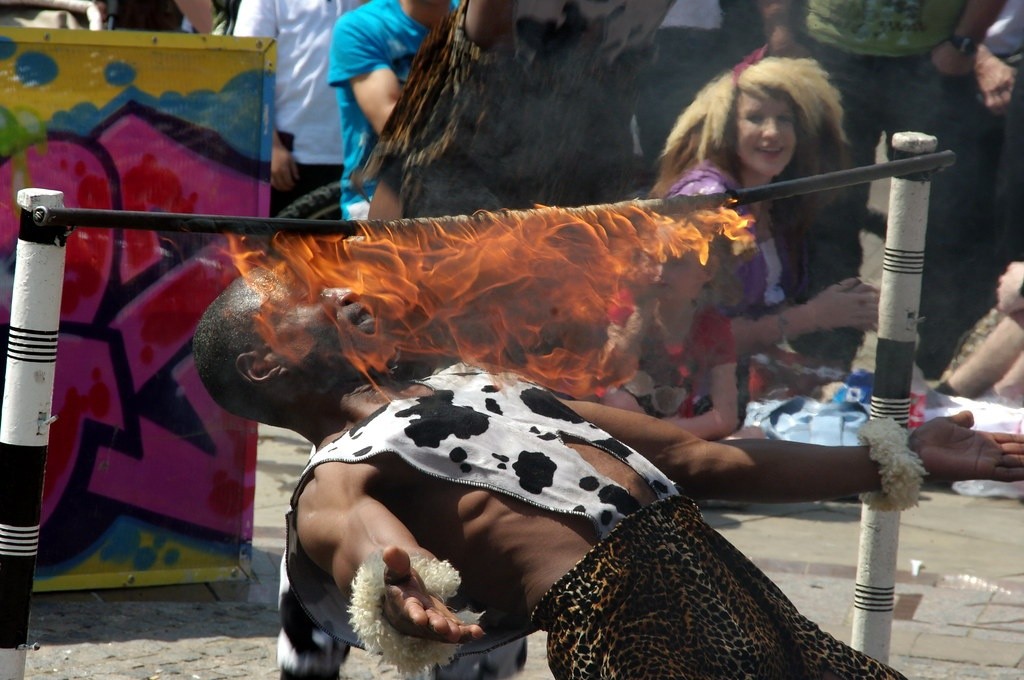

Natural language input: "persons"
[193,264,1024,680]
[0,0,1024,438]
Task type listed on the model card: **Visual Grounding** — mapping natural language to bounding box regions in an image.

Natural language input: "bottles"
[908,363,927,431]
